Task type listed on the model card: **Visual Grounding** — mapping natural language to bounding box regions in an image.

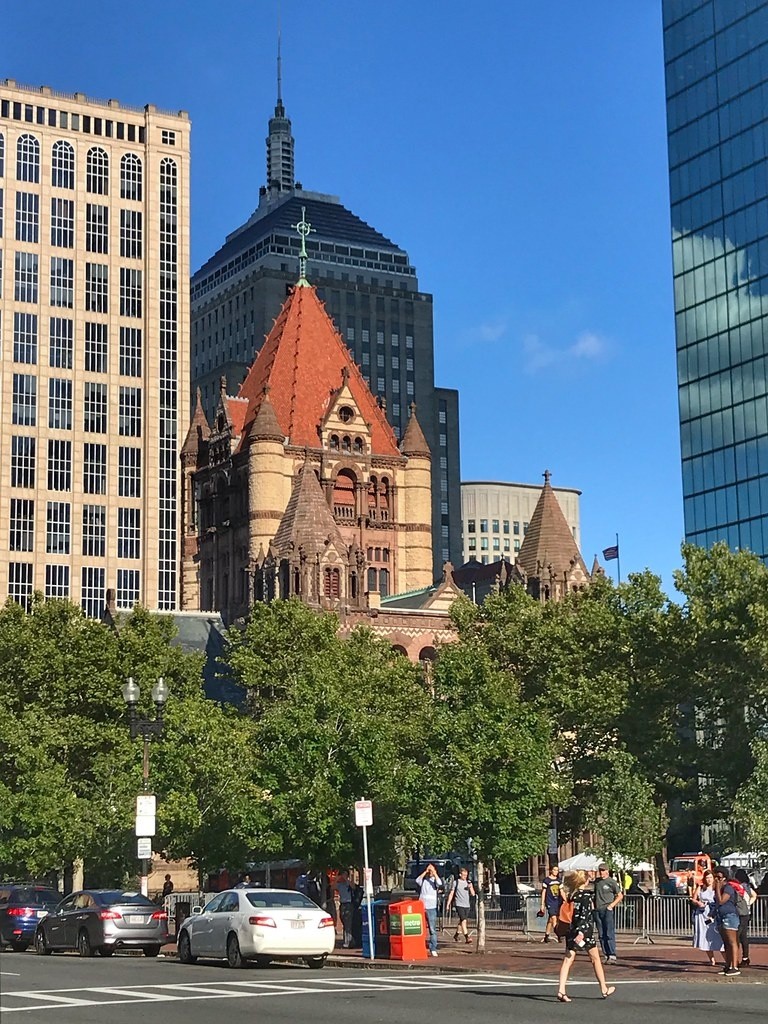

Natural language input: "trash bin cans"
[361,899,428,961]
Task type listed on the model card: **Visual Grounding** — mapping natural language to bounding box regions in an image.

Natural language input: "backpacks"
[728,884,750,917]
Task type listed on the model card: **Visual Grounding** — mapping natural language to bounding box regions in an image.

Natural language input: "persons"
[162,874,173,898]
[686,865,758,977]
[235,874,255,888]
[662,875,677,895]
[415,862,442,957]
[296,866,357,948]
[541,864,633,1002]
[446,868,475,943]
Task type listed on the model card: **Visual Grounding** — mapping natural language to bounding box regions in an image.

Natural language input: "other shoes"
[466,938,473,943]
[601,957,617,965]
[726,968,741,976]
[706,961,717,967]
[431,951,438,957]
[555,936,563,943]
[453,935,461,942]
[717,967,731,975]
[544,936,551,944]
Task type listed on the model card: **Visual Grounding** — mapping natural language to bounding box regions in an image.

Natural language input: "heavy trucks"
[404,859,525,918]
[661,852,768,896]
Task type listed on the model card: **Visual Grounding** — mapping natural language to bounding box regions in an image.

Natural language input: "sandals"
[601,987,616,999]
[557,992,571,1003]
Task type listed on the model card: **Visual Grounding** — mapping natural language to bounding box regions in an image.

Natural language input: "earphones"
[460,874,461,876]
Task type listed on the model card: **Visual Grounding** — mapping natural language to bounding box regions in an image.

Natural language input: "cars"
[34,889,167,957]
[177,889,336,969]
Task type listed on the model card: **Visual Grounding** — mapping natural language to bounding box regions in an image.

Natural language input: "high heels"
[739,958,751,967]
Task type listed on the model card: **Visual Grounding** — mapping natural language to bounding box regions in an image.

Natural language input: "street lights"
[120,678,169,899]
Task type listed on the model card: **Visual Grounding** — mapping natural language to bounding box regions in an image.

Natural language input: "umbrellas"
[558,852,654,871]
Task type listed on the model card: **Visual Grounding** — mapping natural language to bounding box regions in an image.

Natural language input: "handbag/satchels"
[688,899,701,910]
[555,900,574,936]
[349,883,364,909]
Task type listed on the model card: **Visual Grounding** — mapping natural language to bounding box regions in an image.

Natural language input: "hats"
[599,864,609,870]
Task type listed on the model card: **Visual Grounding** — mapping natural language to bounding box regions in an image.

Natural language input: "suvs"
[0,880,64,953]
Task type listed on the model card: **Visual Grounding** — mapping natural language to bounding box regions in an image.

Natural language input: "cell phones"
[574,939,586,947]
